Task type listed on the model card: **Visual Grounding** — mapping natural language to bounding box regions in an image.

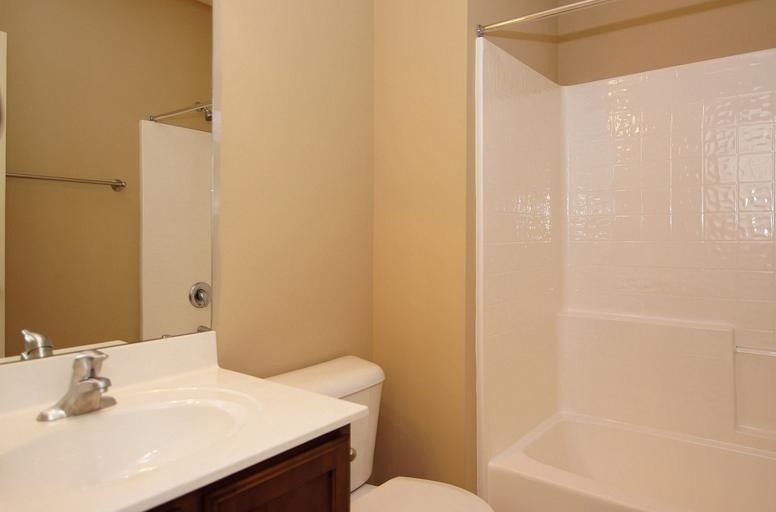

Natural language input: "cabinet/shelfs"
[149,423,351,512]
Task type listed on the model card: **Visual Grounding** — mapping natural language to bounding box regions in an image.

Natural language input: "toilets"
[260,354,493,512]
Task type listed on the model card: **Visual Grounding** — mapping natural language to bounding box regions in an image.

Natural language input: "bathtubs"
[488,411,776,512]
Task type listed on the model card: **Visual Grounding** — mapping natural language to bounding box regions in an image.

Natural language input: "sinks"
[0,383,267,511]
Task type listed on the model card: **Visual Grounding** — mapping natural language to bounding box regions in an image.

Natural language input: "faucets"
[19,328,52,359]
[36,347,115,421]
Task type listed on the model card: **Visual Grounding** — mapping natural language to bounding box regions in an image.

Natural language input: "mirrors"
[0,0,218,368]
